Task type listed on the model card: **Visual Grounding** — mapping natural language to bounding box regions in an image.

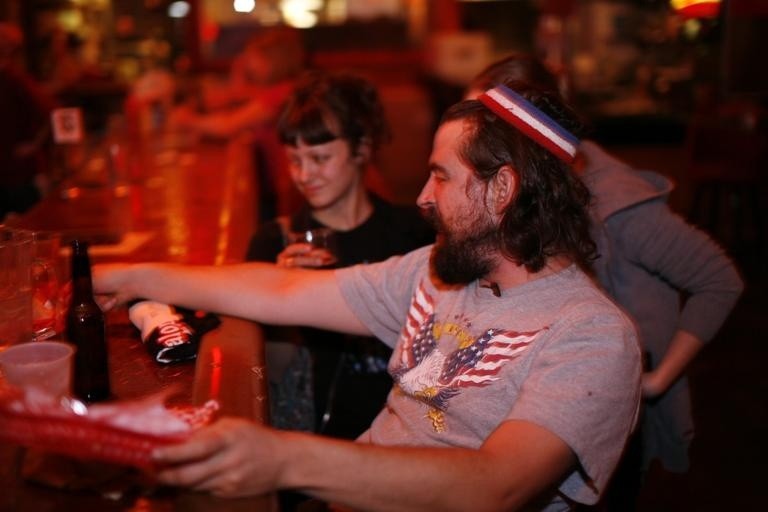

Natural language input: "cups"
[286,227,337,268]
[1,343,75,402]
[0,225,56,349]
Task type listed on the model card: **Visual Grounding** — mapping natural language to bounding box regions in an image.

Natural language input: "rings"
[286,256,294,268]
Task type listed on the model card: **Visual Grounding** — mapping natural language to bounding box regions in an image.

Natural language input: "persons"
[56,86,643,510]
[241,78,438,443]
[460,55,742,509]
[1,0,767,234]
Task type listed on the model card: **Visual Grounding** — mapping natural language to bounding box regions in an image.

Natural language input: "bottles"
[67,240,110,398]
